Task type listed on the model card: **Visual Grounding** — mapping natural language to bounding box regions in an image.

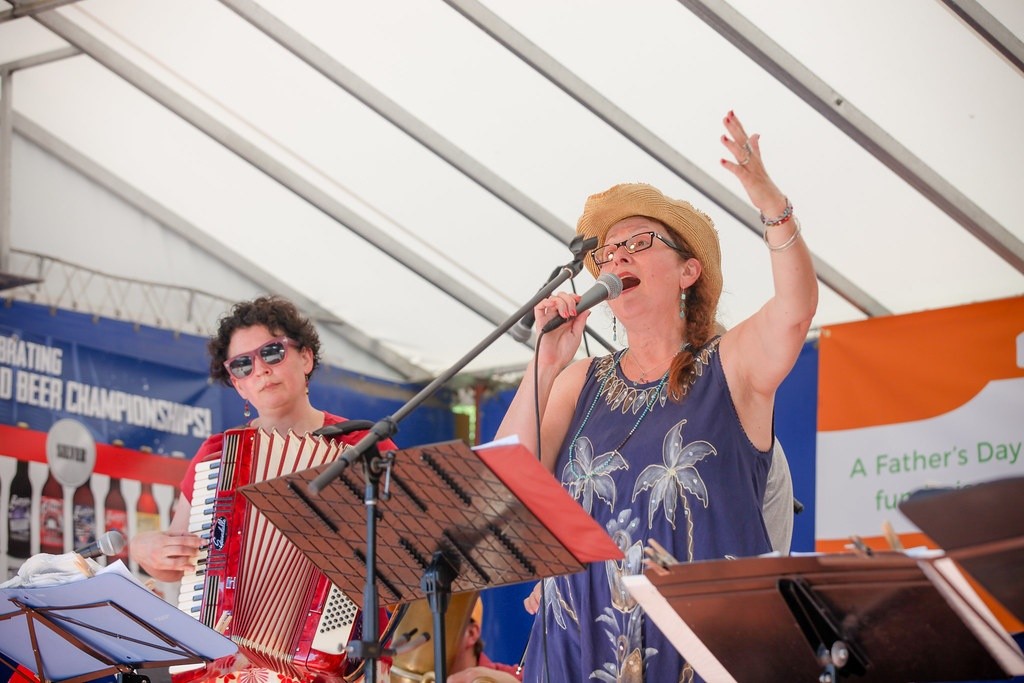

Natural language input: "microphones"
[541,272,623,332]
[74,531,125,559]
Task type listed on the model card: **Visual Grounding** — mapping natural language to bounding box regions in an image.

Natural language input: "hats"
[575,182,722,325]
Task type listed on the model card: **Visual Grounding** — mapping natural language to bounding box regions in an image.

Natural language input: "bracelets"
[760,197,793,226]
[763,215,801,252]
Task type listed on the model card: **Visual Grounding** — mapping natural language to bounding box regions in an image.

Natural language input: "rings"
[743,140,753,153]
[741,157,751,165]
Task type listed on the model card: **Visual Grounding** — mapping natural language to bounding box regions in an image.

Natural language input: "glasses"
[223,334,301,380]
[591,230,684,271]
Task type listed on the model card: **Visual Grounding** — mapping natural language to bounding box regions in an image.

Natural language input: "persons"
[477,111,818,683]
[447,617,525,683]
[132,295,397,683]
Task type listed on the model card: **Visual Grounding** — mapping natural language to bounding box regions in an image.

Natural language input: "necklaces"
[628,350,673,384]
[568,343,691,479]
